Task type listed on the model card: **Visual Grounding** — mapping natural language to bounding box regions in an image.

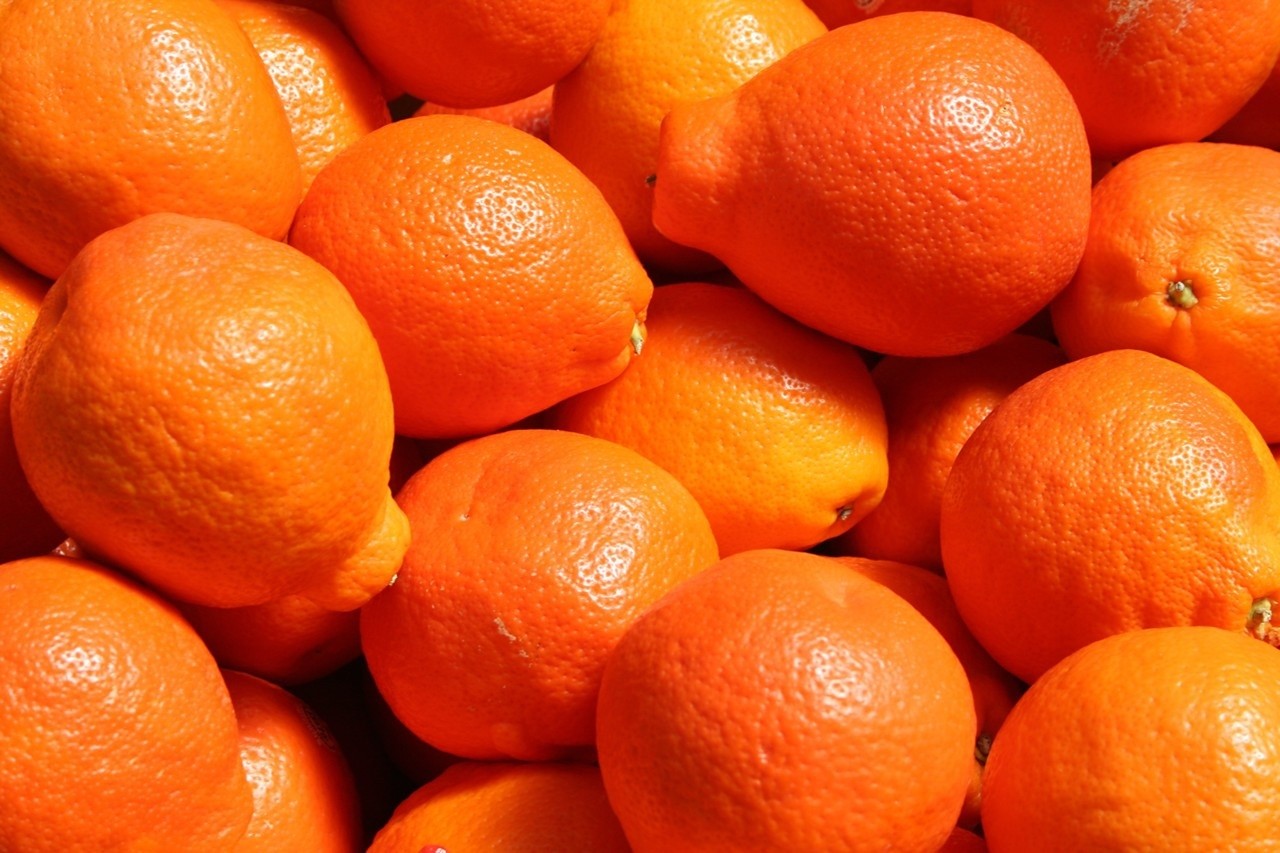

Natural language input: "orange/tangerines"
[1,2,1278,849]
